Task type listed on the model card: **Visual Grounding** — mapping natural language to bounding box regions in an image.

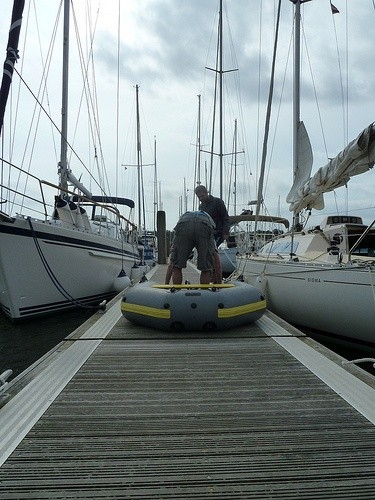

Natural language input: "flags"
[331,3,339,14]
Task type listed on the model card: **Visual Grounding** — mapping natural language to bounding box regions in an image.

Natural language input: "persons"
[195,186,231,284]
[165,211,221,290]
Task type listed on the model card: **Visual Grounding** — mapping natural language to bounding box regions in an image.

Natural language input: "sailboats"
[191,1,374,347]
[0,0,158,327]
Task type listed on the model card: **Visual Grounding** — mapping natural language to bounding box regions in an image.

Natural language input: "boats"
[120,278,267,332]
